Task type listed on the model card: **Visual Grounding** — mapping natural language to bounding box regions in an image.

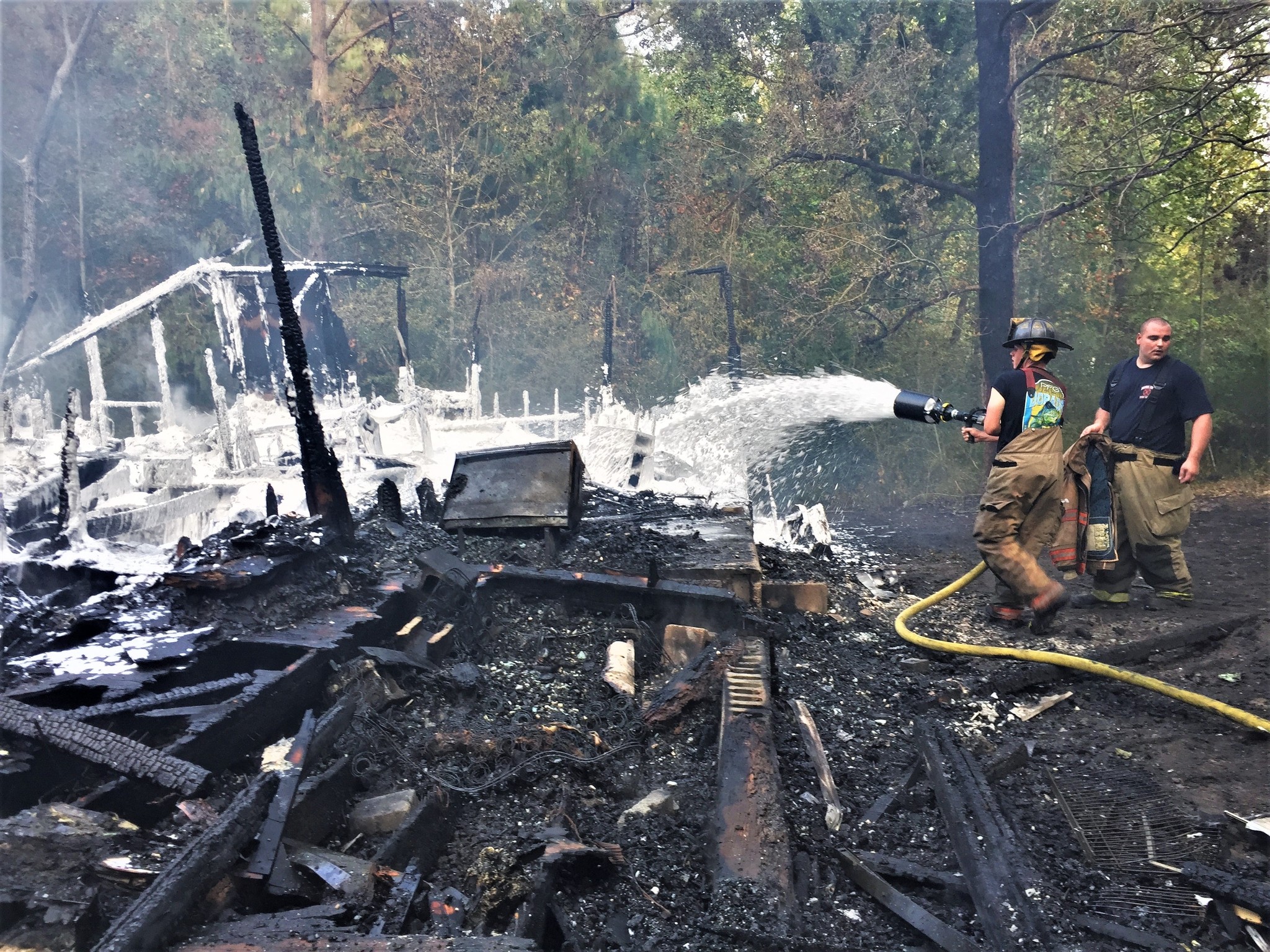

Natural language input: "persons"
[960,318,1075,636]
[1077,316,1215,611]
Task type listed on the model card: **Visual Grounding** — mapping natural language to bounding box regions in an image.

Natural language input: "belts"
[1110,453,1174,467]
[992,459,1017,469]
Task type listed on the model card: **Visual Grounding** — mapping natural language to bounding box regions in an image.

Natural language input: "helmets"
[1002,317,1075,351]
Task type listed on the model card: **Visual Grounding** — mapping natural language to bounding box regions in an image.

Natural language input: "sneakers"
[1068,594,1126,610]
[1143,597,1191,609]
[1028,589,1070,636]
[984,606,1026,628]
